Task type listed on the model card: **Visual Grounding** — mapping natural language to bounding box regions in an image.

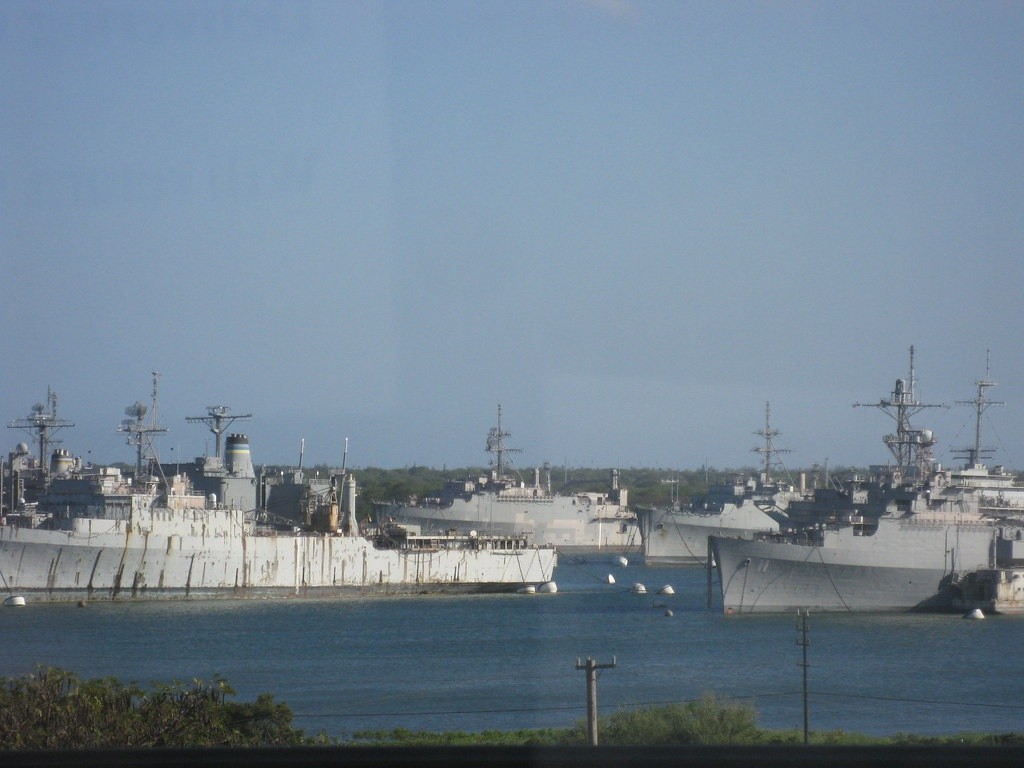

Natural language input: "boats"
[0,368,1024,617]
